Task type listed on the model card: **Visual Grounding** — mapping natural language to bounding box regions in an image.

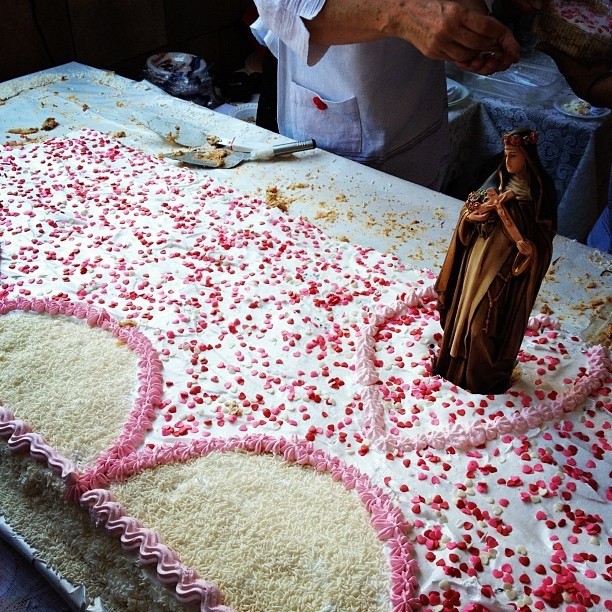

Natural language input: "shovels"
[164,138,316,168]
[131,111,292,158]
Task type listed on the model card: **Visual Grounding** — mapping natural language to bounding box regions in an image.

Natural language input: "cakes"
[565,97,592,115]
[0,130,610,606]
[533,1,612,61]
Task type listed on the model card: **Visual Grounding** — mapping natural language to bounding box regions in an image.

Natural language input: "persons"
[431,128,558,393]
[557,117,611,257]
[250,1,542,195]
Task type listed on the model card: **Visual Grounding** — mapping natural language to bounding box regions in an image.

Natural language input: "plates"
[553,93,612,118]
